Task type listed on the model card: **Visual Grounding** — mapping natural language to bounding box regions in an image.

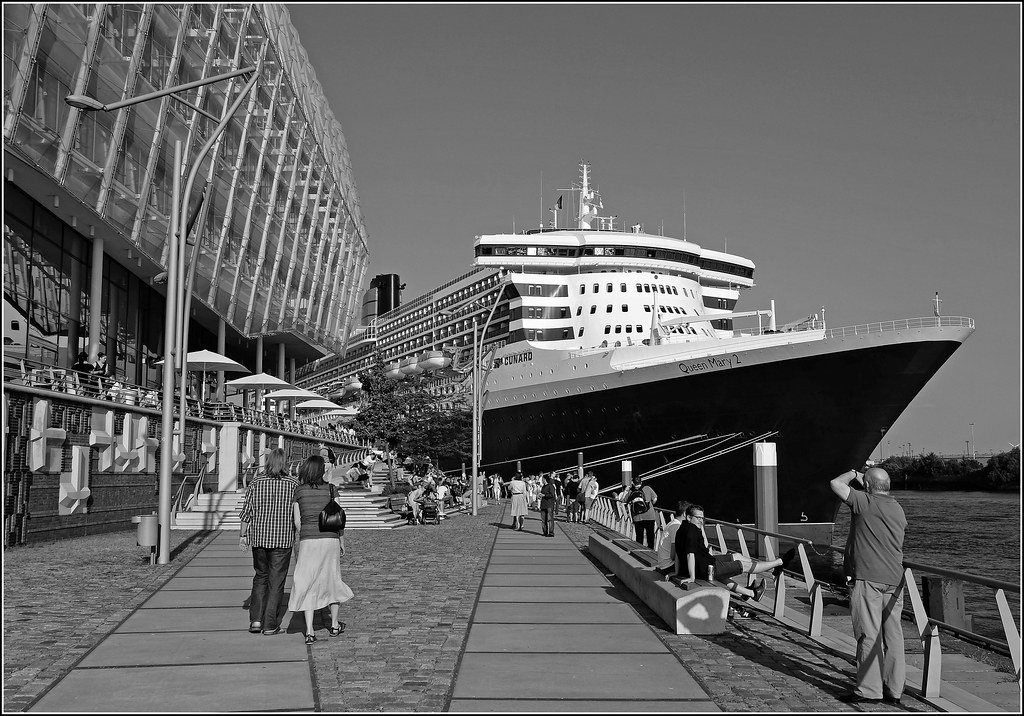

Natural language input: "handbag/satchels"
[318,483,346,532]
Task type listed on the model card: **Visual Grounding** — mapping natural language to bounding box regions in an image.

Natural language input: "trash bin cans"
[131,515,157,548]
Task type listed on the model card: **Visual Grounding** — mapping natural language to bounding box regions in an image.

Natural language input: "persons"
[238,448,303,635]
[205,399,337,436]
[831,468,908,703]
[317,441,336,483]
[346,450,599,537]
[287,455,354,645]
[70,352,117,399]
[612,476,796,602]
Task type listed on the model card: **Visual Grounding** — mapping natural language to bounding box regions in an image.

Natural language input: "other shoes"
[842,691,900,705]
[249,621,285,635]
[753,578,766,602]
[542,533,554,537]
[774,548,796,572]
[577,492,585,503]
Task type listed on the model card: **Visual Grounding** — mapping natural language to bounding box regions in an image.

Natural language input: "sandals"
[304,634,316,645]
[330,621,346,636]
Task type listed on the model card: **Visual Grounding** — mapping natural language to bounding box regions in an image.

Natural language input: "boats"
[417,351,452,371]
[383,364,405,381]
[316,376,362,401]
[399,357,425,376]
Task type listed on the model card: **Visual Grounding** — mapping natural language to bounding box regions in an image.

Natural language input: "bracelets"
[852,469,858,477]
[241,534,247,537]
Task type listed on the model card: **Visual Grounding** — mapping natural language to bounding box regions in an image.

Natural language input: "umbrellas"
[154,350,252,419]
[224,373,347,427]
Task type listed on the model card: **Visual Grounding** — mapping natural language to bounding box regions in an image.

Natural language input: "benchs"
[390,493,413,518]
[588,529,731,635]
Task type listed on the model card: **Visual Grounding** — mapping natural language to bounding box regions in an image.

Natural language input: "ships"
[288,158,976,569]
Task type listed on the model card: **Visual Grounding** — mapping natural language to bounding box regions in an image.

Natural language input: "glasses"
[691,515,704,520]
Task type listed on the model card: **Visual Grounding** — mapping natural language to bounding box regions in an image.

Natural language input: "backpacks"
[629,485,651,515]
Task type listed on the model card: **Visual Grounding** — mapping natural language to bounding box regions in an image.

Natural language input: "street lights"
[439,281,508,515]
[59,55,264,568]
